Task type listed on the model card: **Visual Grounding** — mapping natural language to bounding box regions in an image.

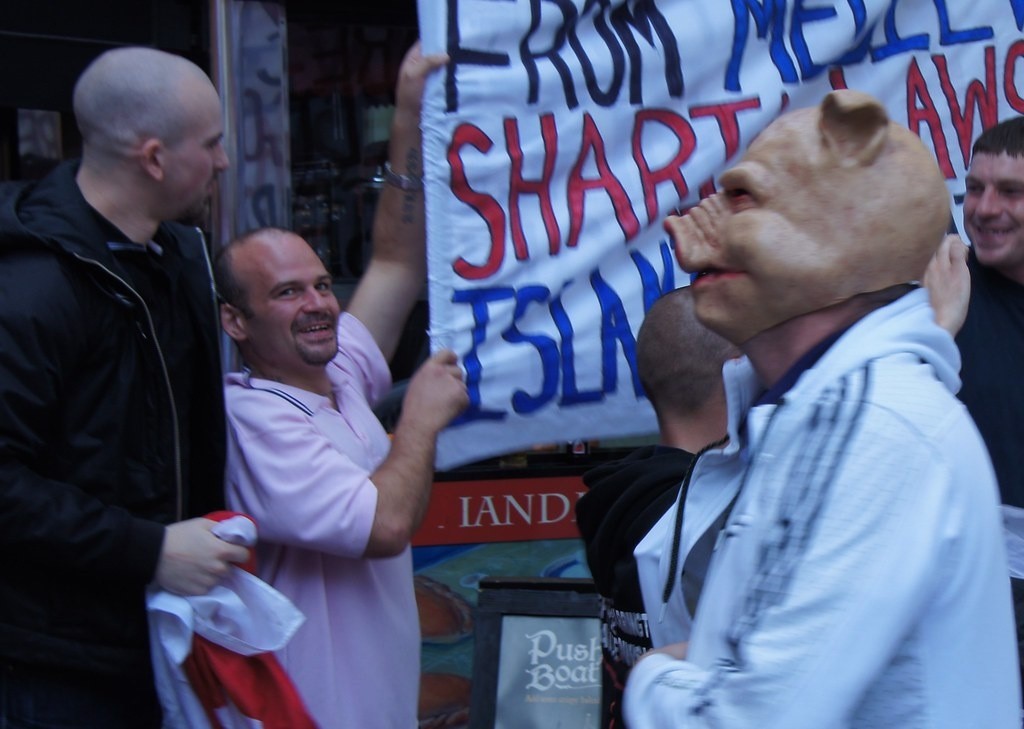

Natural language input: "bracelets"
[383,163,425,191]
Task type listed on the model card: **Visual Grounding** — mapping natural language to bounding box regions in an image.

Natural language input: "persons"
[619,89,1021,728]
[572,232,981,729]
[212,36,467,728]
[957,111,1023,665]
[0,45,253,728]
